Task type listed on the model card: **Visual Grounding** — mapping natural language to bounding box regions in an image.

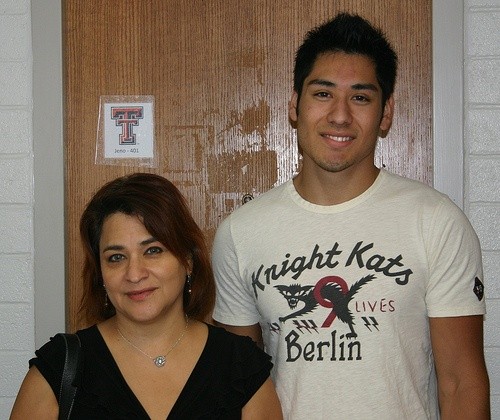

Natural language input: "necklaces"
[111,317,189,367]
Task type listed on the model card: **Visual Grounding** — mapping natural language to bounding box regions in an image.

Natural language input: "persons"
[212,12,491,420]
[9,172,283,420]
